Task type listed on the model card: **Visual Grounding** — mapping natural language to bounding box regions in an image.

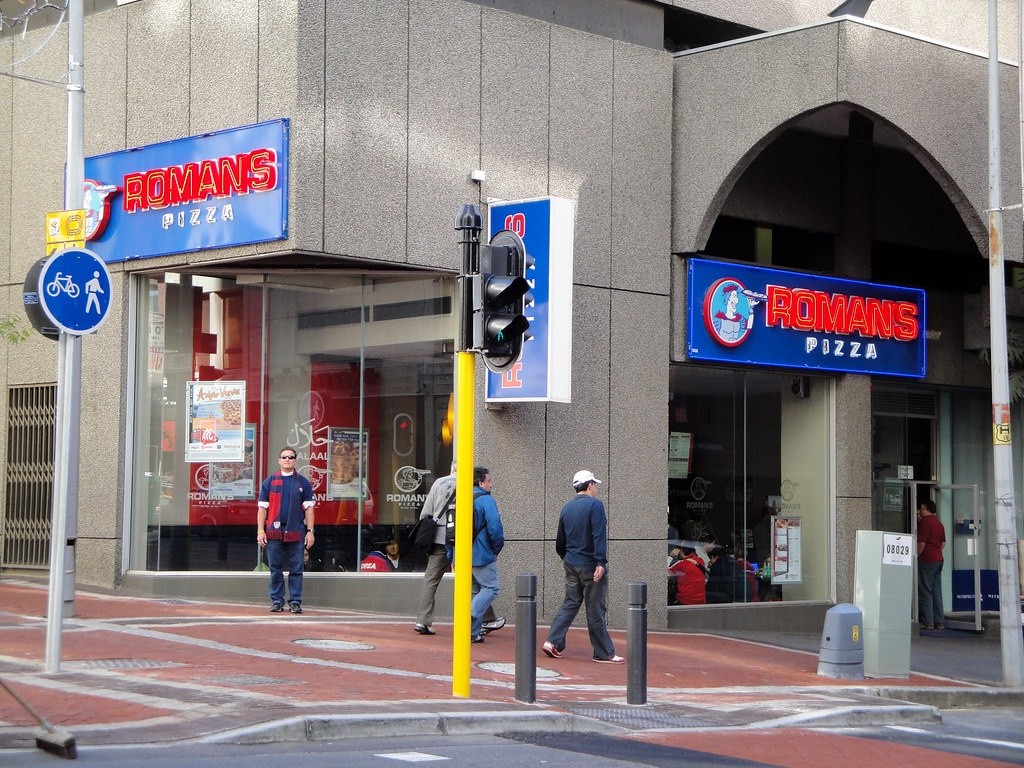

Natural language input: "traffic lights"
[395,415,415,455]
[490,229,534,350]
[479,272,530,362]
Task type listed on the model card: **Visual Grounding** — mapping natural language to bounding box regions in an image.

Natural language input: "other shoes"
[919,624,934,630]
[935,623,945,629]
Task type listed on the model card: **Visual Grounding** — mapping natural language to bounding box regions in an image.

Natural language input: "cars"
[667,539,725,580]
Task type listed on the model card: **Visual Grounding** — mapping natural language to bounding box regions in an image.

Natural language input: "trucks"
[191,368,379,532]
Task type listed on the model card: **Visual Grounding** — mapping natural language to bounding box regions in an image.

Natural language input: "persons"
[444,465,505,643]
[413,458,506,635]
[667,504,782,606]
[257,447,316,613]
[542,469,625,665]
[253,532,412,572]
[919,500,945,629]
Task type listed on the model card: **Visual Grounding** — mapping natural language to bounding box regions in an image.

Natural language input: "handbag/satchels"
[407,514,438,555]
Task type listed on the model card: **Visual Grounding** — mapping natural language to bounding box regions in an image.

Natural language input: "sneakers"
[471,634,484,643]
[414,623,436,635]
[269,600,284,612]
[479,616,506,636]
[542,641,565,659]
[592,655,625,665]
[288,603,303,613]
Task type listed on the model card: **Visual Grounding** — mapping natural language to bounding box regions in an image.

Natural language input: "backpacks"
[445,492,491,546]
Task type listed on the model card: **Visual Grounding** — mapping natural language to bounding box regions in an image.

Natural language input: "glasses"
[278,455,295,460]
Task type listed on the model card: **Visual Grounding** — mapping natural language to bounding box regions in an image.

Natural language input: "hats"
[573,469,602,487]
